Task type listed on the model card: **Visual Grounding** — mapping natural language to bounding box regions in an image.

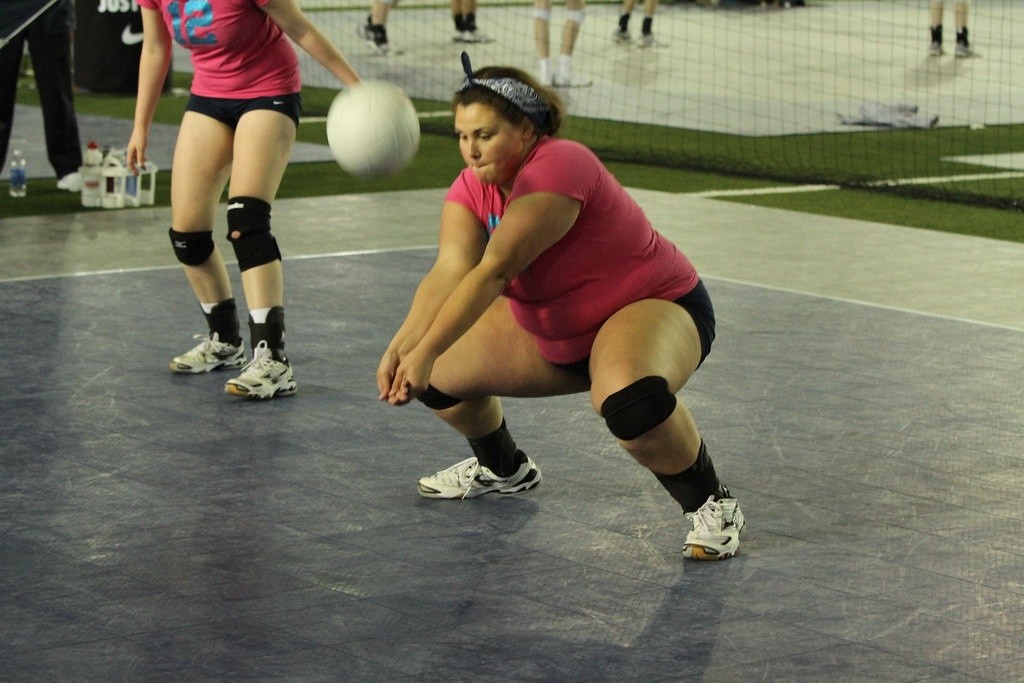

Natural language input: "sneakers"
[359,16,393,56]
[554,70,594,87]
[224,340,296,398]
[540,71,556,88]
[681,493,745,559]
[416,449,542,498]
[168,331,247,373]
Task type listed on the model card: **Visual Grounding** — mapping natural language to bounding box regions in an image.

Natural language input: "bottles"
[80,141,104,207]
[8,149,26,198]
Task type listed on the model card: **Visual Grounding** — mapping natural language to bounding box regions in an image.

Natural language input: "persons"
[447,0,493,44]
[927,1,982,60]
[356,1,391,53]
[529,1,595,89]
[126,1,360,400]
[373,66,743,561]
[612,1,665,46]
[0,0,83,192]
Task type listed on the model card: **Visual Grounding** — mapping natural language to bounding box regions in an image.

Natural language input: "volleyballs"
[326,80,420,175]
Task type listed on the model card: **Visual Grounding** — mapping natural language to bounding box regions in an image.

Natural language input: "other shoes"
[56,171,82,193]
[464,29,488,42]
[930,42,943,56]
[452,30,464,41]
[612,30,635,47]
[637,34,669,49]
[955,44,973,57]
